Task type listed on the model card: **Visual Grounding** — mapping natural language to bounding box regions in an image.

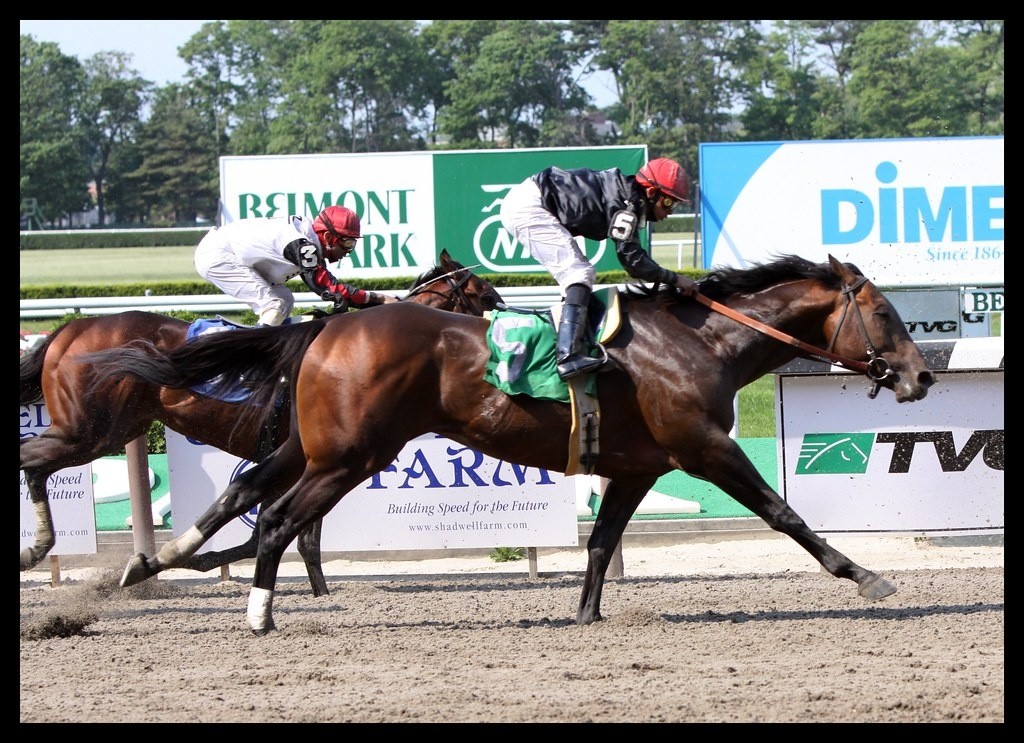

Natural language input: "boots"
[553,303,614,381]
[236,322,277,388]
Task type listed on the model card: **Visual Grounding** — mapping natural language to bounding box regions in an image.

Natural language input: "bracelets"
[664,270,678,285]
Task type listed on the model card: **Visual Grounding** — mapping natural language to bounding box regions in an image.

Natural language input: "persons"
[497,157,702,383]
[193,205,401,327]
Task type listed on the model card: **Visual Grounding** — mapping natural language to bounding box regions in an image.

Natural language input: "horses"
[18,249,939,639]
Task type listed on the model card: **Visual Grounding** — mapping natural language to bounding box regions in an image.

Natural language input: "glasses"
[335,235,357,254]
[662,193,681,212]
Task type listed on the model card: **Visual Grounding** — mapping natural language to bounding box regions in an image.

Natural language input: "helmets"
[313,205,365,238]
[635,157,693,204]
